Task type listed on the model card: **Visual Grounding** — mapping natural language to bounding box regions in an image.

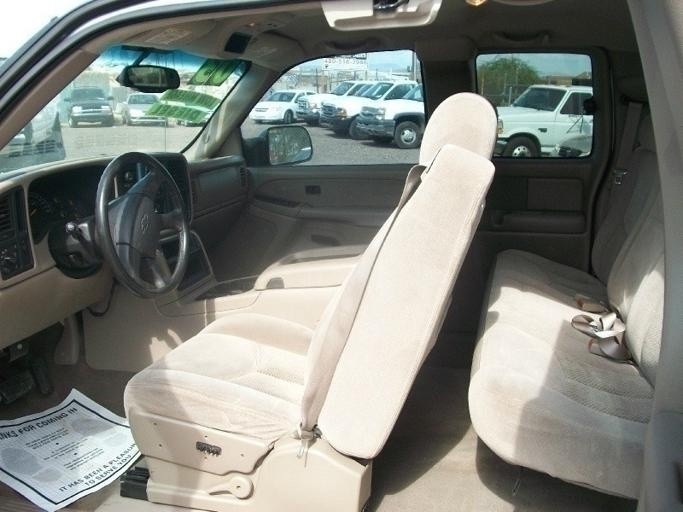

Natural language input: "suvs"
[0,56,233,174]
[319,79,421,141]
[295,80,374,126]
[494,83,594,160]
[355,83,425,150]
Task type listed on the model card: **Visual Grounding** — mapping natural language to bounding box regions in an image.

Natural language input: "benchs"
[465,108,665,499]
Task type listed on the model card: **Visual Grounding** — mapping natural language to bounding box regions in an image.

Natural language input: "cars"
[249,90,319,125]
[549,135,592,158]
